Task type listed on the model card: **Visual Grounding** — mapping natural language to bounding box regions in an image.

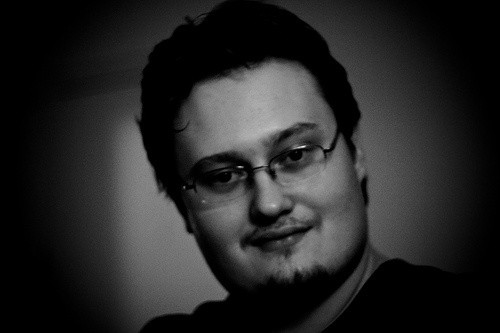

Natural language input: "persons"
[131,1,500,333]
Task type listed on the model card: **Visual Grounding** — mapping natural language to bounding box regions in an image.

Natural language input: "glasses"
[176,124,342,205]
